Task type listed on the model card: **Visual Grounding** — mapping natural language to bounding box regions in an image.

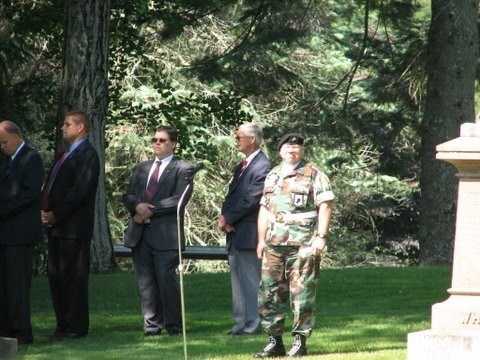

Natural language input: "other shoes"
[18,335,32,344]
[226,331,259,336]
[287,333,307,357]
[254,335,286,357]
[52,329,86,340]
[145,329,181,336]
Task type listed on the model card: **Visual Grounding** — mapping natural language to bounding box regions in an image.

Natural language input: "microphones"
[184,163,204,181]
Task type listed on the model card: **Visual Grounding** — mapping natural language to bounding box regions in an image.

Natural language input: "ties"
[145,161,162,203]
[8,157,12,166]
[239,161,246,176]
[42,149,69,208]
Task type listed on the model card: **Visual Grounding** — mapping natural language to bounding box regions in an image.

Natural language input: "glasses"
[236,136,248,140]
[151,138,171,141]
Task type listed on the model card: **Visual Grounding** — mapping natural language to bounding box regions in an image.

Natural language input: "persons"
[122,125,193,336]
[40,110,100,339]
[216,122,273,336]
[0,120,45,344]
[253,133,335,358]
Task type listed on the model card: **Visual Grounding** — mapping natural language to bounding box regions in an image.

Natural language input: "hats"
[277,133,303,148]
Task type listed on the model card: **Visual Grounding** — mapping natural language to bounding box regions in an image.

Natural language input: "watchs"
[315,232,327,239]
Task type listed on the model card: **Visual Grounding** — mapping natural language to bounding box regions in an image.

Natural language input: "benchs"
[109,242,228,260]
